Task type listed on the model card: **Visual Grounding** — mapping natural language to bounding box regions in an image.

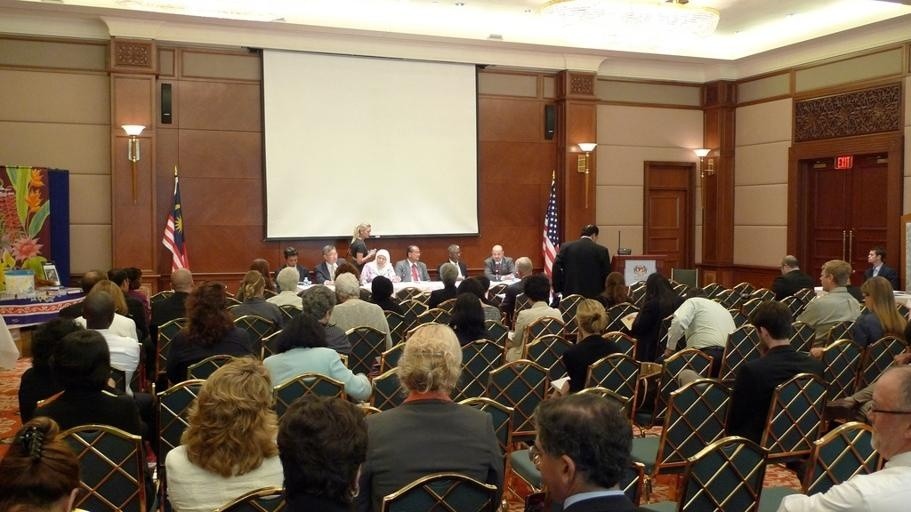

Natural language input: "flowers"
[0,166,50,279]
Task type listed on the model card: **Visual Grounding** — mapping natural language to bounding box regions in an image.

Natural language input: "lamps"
[576,141,599,208]
[119,123,145,205]
[693,147,711,208]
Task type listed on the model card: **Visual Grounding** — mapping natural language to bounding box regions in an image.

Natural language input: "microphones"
[495,261,501,280]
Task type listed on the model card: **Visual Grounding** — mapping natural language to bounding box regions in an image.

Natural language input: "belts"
[700,346,725,353]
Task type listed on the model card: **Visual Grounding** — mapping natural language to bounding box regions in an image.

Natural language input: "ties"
[412,263,419,282]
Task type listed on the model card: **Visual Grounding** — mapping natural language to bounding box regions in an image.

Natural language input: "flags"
[542,179,560,279]
[162,176,189,272]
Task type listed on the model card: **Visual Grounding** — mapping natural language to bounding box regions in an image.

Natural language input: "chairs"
[62,425,146,512]
[149,266,910,512]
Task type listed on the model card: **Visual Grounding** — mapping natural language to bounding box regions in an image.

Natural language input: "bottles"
[494,270,501,281]
[302,277,310,289]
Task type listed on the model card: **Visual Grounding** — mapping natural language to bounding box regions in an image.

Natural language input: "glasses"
[528,445,561,464]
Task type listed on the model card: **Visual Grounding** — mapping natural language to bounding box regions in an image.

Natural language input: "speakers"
[160,84,173,125]
[545,105,556,139]
